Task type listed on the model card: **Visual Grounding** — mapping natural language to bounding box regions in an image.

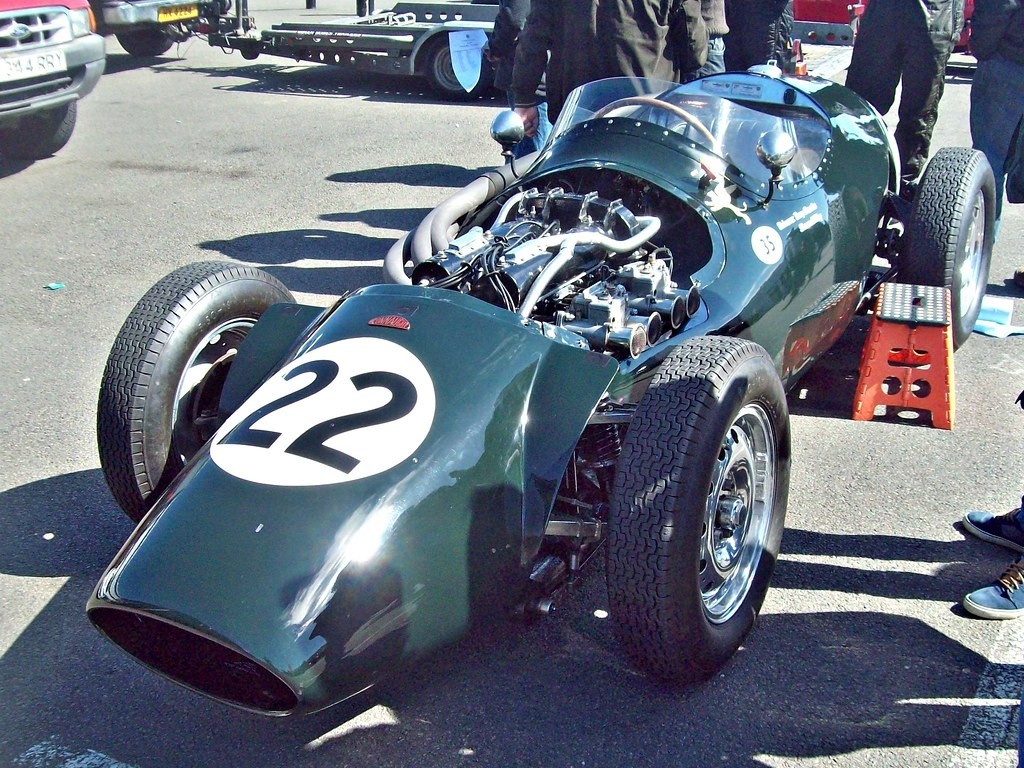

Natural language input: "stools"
[851,282,954,431]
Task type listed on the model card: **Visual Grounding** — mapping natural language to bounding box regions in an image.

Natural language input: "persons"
[967,0,1024,243]
[482,0,797,165]
[845,0,965,226]
[962,496,1024,620]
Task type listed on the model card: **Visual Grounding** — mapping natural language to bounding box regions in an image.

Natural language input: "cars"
[85,39,998,719]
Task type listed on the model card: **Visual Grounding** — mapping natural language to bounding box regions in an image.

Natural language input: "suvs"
[0,0,106,157]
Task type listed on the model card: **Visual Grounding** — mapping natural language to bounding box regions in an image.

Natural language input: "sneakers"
[963,495,1024,551]
[963,555,1024,621]
